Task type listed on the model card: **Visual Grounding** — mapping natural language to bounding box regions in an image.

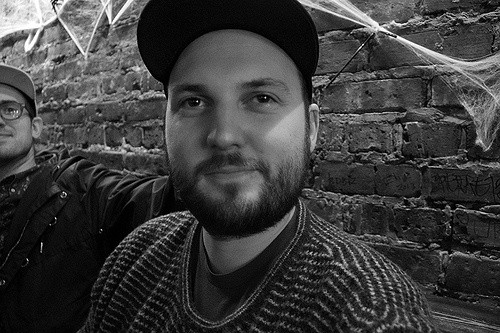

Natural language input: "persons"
[0,64,187,332]
[77,0,439,332]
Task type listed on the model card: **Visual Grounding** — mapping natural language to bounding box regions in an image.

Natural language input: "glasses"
[0,101,33,121]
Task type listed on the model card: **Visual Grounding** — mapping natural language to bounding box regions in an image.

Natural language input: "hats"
[137,0,319,104]
[0,64,37,118]
